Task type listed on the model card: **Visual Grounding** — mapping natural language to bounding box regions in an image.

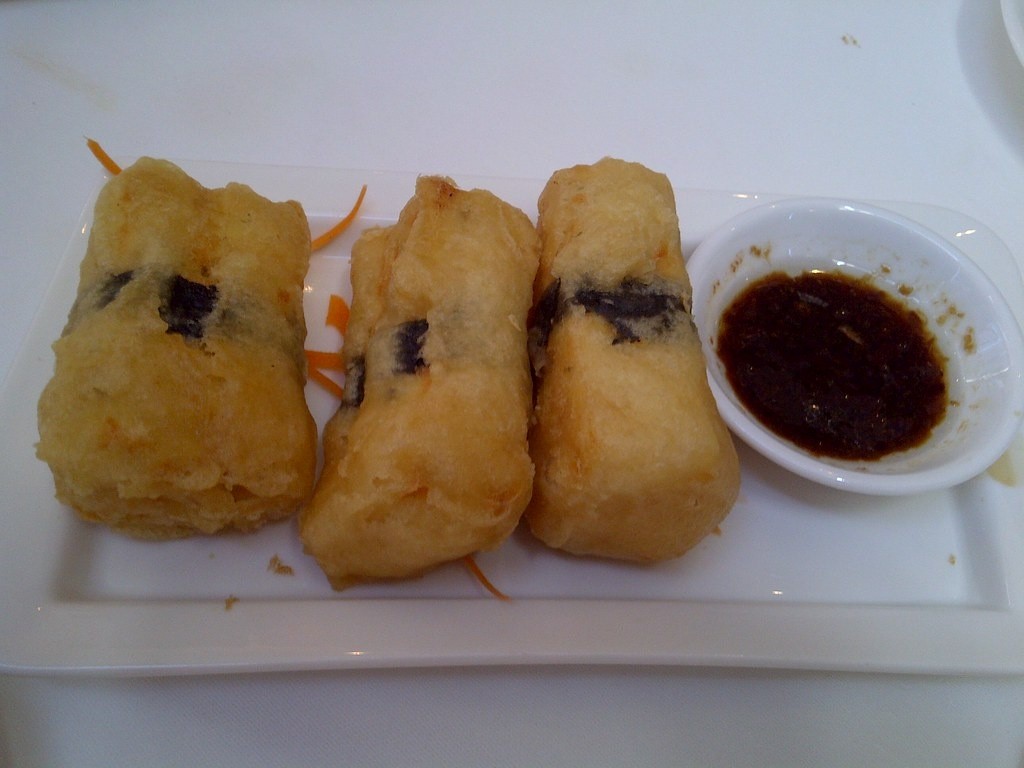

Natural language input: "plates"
[2,156,1024,678]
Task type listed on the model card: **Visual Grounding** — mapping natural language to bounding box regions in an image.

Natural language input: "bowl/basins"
[690,198,1024,494]
